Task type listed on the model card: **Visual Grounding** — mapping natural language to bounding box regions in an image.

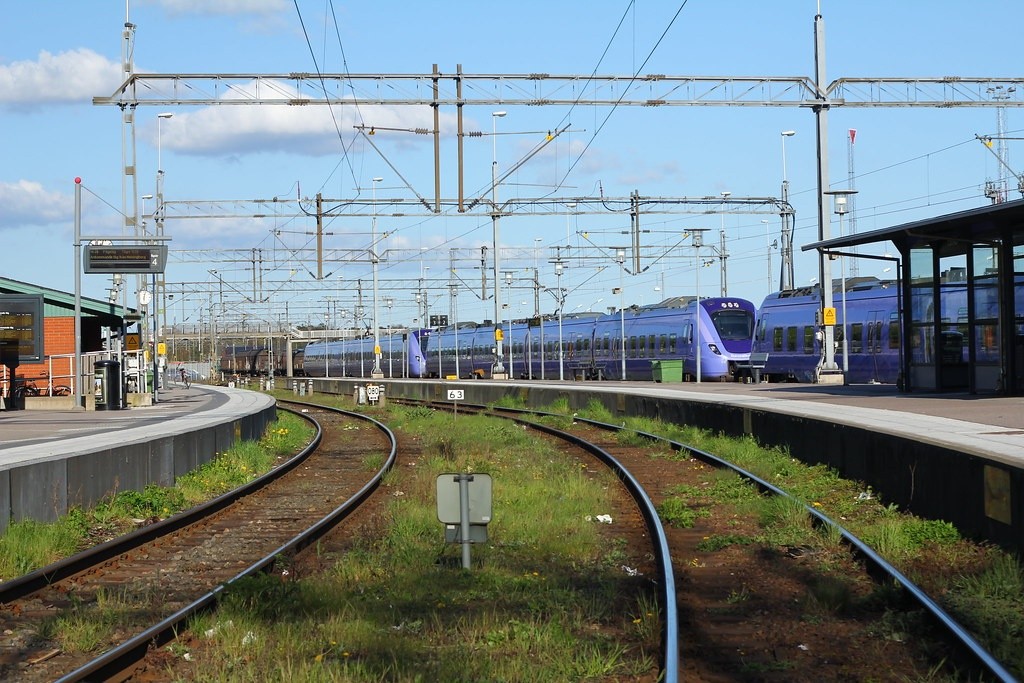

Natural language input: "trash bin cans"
[147,370,154,394]
[650,359,683,384]
[93,359,120,411]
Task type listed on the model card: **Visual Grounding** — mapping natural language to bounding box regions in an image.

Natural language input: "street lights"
[565,199,579,262]
[762,219,774,294]
[420,247,432,329]
[492,110,512,381]
[370,176,386,378]
[825,189,858,388]
[782,130,796,292]
[719,191,732,298]
[138,194,154,393]
[151,112,174,404]
[533,237,542,323]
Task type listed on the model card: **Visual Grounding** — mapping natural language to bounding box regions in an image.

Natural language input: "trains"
[219,272,1024,394]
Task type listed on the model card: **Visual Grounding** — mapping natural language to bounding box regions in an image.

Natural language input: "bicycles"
[180,368,192,390]
[18,370,72,396]
